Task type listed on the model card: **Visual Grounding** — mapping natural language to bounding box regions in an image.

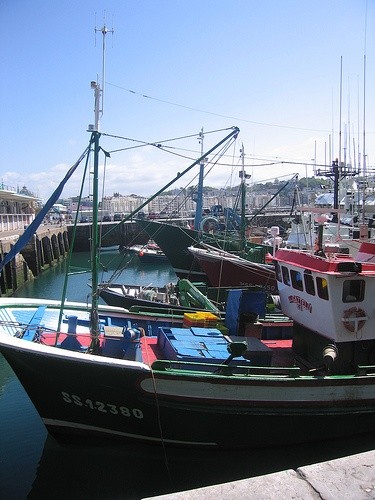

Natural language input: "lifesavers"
[342,305,367,332]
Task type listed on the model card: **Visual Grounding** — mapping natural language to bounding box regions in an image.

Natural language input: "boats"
[85,141,252,314]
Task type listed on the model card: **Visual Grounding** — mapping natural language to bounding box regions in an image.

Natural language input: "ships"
[134,142,304,279]
[0,10,373,456]
